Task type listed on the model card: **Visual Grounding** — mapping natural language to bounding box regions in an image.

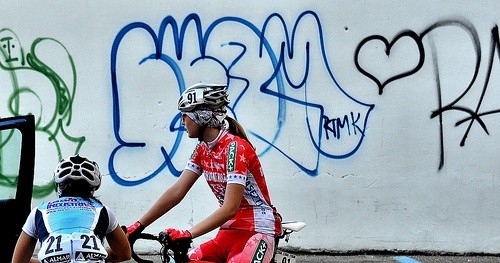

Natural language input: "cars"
[0,115,35,263]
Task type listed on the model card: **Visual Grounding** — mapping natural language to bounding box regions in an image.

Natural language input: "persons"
[12,154,131,263]
[125,83,282,263]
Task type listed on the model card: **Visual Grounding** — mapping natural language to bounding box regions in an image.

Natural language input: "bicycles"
[121,220,308,263]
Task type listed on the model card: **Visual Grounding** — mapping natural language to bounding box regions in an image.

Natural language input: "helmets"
[178,83,229,115]
[54,153,102,191]
